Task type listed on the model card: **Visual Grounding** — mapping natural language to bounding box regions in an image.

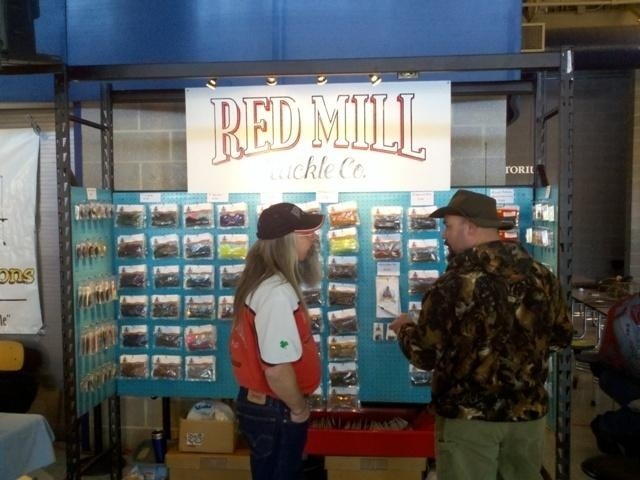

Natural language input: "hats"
[429,190,515,228]
[257,203,325,239]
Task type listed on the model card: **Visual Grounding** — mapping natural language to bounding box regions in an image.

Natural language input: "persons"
[230,203,326,479]
[388,187,572,479]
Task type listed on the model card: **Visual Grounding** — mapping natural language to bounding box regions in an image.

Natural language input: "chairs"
[0,340,25,412]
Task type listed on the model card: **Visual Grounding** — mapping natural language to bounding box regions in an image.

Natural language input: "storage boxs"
[176,401,239,454]
[324,456,428,480]
[304,410,438,459]
[165,444,252,479]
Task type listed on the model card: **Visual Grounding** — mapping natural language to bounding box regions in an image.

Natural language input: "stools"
[572,332,600,409]
[580,455,640,479]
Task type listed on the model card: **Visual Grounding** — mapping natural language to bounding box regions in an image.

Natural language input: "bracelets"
[291,400,307,416]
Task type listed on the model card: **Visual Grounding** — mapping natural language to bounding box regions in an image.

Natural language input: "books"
[313,411,413,432]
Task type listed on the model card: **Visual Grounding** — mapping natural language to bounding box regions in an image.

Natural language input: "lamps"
[203,74,384,89]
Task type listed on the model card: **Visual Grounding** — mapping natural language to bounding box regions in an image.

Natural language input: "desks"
[1,413,57,480]
[572,288,637,350]
